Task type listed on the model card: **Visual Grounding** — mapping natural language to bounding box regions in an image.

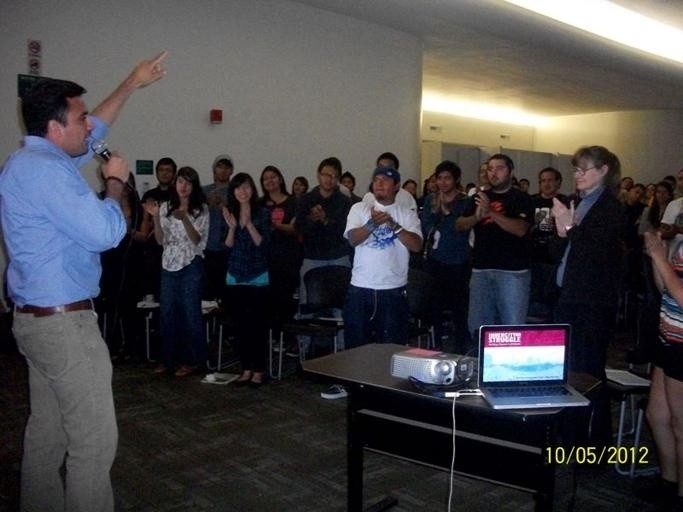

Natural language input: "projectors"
[390,348,474,388]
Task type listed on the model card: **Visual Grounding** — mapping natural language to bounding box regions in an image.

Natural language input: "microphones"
[91,138,132,191]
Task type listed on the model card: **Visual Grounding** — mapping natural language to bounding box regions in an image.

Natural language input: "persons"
[0,49,166,512]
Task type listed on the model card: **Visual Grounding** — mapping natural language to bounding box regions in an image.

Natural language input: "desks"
[297,343,610,512]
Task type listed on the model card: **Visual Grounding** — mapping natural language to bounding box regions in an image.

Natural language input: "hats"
[212,154,234,167]
[373,166,401,182]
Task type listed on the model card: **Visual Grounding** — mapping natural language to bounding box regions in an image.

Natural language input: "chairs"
[95,264,654,381]
[587,358,669,477]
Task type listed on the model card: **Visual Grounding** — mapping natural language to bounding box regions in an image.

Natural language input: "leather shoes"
[234,373,267,387]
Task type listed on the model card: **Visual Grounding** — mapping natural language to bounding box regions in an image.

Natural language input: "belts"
[15,299,95,317]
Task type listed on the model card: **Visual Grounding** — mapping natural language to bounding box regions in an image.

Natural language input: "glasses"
[320,172,338,179]
[572,165,598,177]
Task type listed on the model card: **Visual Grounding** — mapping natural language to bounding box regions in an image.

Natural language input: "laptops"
[478,324,591,409]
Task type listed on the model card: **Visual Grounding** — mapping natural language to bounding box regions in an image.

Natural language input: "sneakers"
[632,475,683,512]
[570,446,606,480]
[152,365,198,377]
[320,383,349,401]
